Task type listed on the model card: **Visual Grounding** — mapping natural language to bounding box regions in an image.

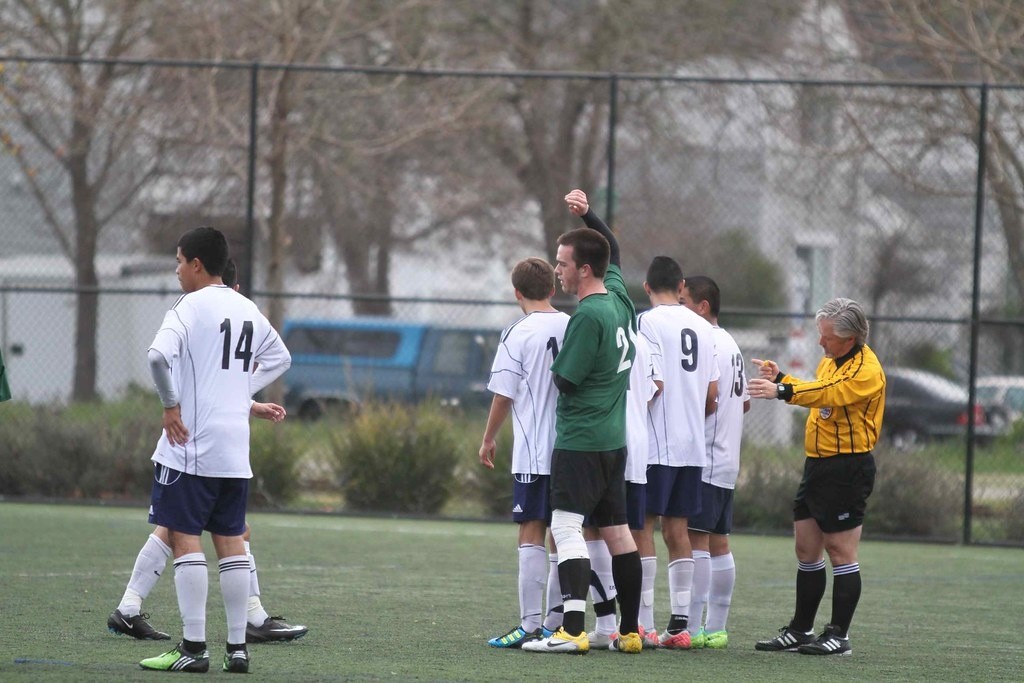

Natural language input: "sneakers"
[245,615,308,644]
[755,620,816,652]
[139,641,209,673]
[108,609,171,641]
[488,625,560,647]
[608,626,644,642]
[522,626,643,653]
[587,629,615,650]
[798,623,852,656]
[692,626,729,649]
[645,630,691,653]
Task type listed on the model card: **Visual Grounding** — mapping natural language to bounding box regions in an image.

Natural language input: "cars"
[877,364,1015,453]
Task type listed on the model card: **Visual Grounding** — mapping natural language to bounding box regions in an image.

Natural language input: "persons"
[108,258,308,646]
[480,188,720,653]
[745,298,887,655]
[678,276,751,648]
[139,226,292,673]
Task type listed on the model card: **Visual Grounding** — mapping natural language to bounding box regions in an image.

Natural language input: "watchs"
[776,383,785,400]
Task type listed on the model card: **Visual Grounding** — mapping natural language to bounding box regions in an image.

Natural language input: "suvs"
[963,374,1024,424]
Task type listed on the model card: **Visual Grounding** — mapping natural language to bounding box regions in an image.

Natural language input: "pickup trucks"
[281,315,505,420]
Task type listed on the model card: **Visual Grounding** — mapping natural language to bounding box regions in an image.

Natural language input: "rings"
[760,389,762,394]
